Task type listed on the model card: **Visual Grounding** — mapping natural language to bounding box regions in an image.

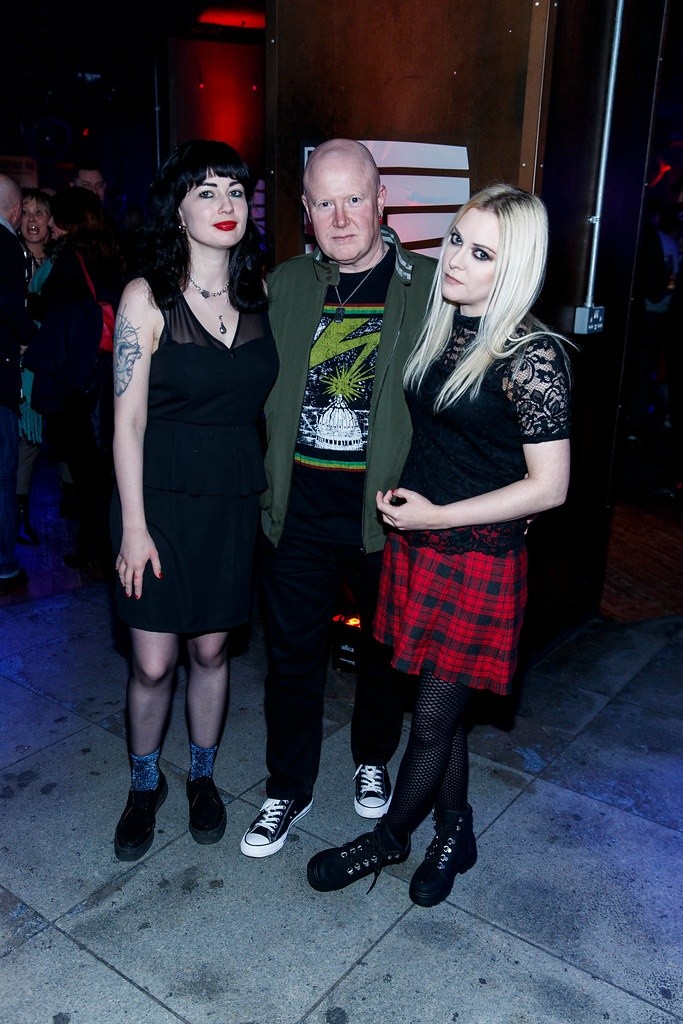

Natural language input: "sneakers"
[185,766,226,846]
[240,791,314,856]
[353,761,392,820]
[114,767,167,861]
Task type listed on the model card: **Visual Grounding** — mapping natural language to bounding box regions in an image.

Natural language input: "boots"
[300,818,412,895]
[14,494,41,545]
[408,805,475,907]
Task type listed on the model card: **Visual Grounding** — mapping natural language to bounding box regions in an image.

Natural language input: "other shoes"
[1,570,28,593]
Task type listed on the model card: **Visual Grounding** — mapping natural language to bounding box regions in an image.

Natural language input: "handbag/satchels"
[21,294,116,395]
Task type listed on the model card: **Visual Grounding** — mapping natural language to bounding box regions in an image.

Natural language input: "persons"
[307,182,572,906]
[0,163,128,595]
[112,139,280,862]
[239,137,440,858]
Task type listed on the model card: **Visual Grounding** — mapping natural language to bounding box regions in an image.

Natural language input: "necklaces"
[186,274,233,336]
[333,246,387,323]
